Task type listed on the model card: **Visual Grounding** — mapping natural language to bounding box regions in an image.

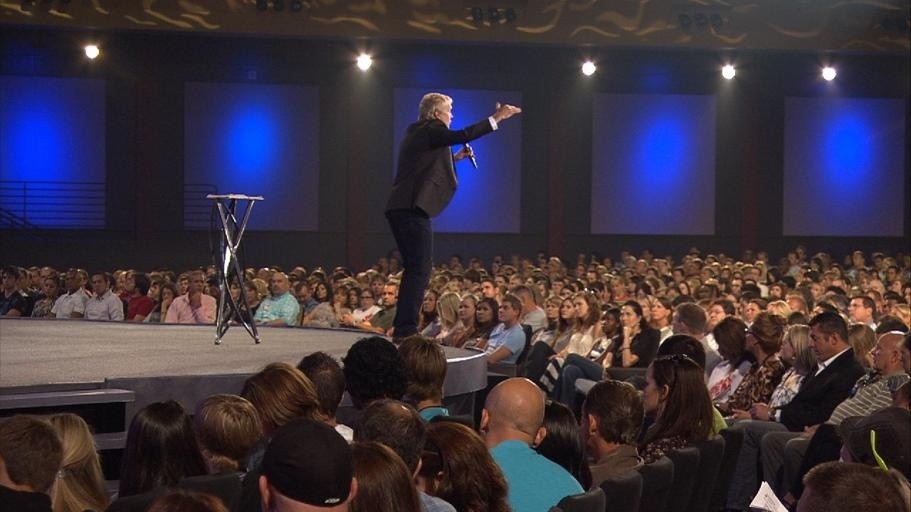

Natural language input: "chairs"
[546,428,742,512]
[516,324,532,363]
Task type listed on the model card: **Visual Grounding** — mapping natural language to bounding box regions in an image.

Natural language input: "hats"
[260,420,351,507]
[840,407,911,473]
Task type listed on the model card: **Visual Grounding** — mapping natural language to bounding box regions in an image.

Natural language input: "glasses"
[743,327,758,338]
[670,354,687,389]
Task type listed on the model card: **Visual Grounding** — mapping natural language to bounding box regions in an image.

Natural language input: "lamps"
[471,7,517,24]
[251,0,304,14]
[679,12,724,30]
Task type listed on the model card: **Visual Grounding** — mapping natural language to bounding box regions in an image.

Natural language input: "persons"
[382,93,523,344]
[1,246,911,512]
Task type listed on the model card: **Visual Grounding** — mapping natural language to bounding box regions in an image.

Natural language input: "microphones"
[463,142,477,168]
